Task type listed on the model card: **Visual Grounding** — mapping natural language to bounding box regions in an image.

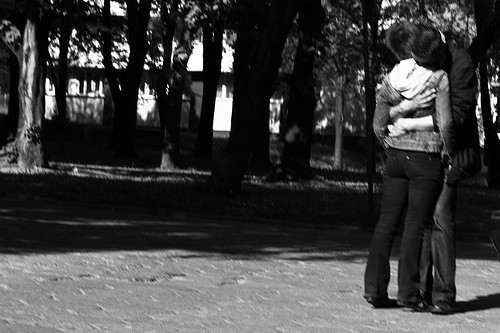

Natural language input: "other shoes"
[365,294,398,309]
[431,298,456,316]
[397,292,429,314]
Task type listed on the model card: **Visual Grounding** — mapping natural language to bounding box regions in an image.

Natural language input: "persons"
[384,26,477,315]
[363,21,453,309]
